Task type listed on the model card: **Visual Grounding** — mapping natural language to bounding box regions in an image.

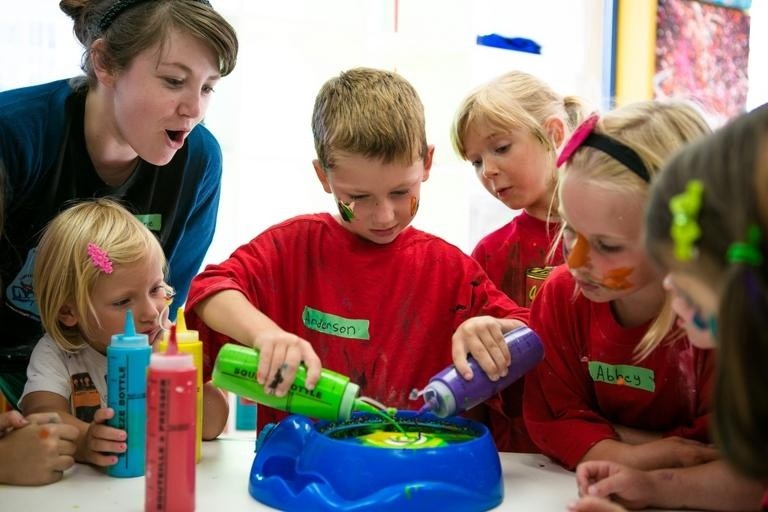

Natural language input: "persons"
[0,407,79,487]
[568,106,768,511]
[451,68,568,458]
[521,99,724,474]
[182,67,533,445]
[14,198,230,467]
[0,0,238,392]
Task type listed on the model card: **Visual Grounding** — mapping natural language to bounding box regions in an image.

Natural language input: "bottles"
[105,303,204,512]
[414,325,548,421]
[209,341,362,424]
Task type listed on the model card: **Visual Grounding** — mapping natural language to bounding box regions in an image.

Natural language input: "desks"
[0,430,582,512]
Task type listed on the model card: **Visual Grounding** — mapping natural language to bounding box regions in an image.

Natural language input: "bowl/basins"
[247,409,507,512]
